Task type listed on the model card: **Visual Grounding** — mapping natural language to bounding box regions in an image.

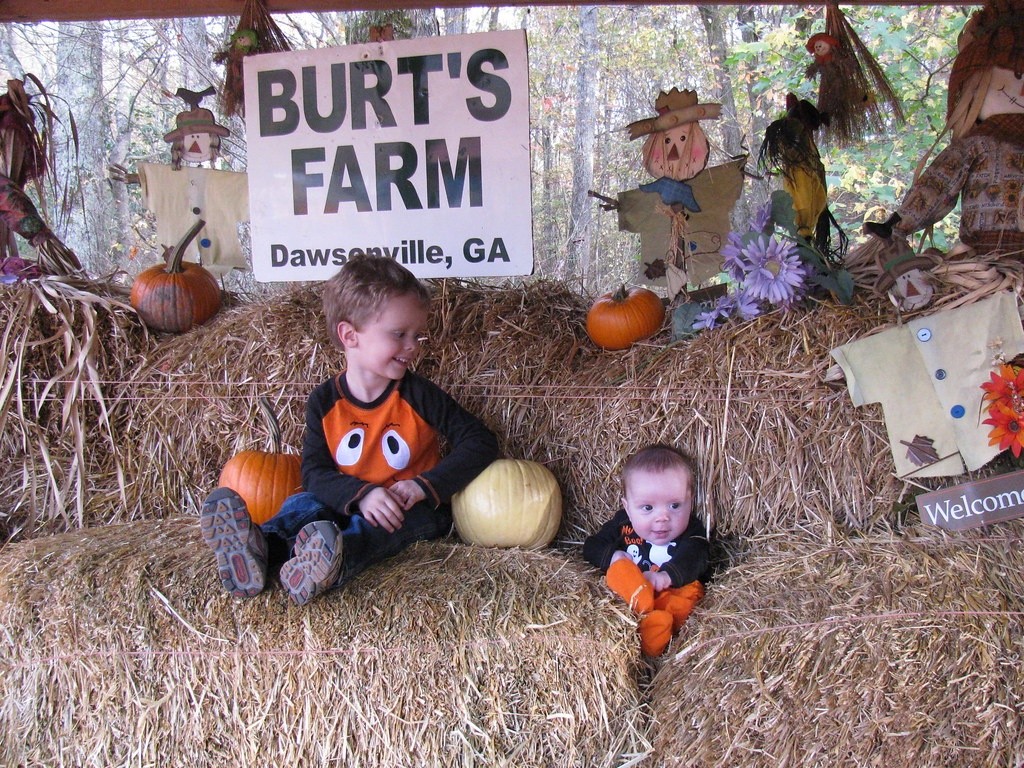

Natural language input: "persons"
[582,444,714,658]
[862,0,1024,272]
[822,212,1024,478]
[803,32,849,95]
[199,256,501,607]
[110,87,251,278]
[588,88,764,300]
[0,74,83,280]
[215,28,259,93]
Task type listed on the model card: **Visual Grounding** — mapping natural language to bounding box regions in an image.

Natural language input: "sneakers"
[279,520,343,606]
[200,487,269,599]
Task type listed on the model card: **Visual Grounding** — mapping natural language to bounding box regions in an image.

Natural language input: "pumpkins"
[218,394,306,526]
[586,284,663,351]
[131,220,221,335]
[451,459,563,549]
